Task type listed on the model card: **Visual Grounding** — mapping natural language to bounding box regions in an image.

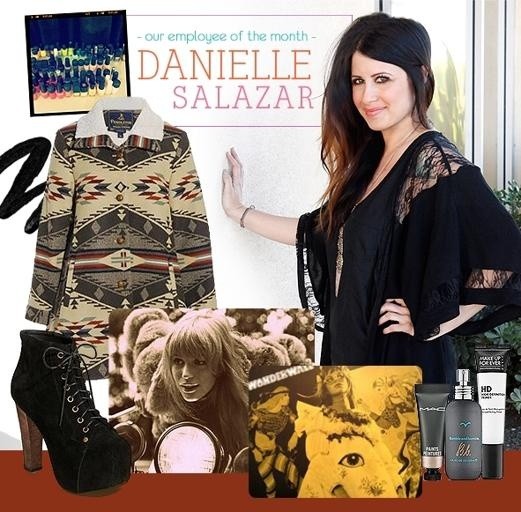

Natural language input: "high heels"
[9,328,132,499]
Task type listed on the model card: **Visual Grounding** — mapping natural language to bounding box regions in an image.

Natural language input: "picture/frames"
[21,9,131,117]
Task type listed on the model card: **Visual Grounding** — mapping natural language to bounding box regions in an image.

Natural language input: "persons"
[218,8,520,385]
[120,308,308,451]
[288,366,406,499]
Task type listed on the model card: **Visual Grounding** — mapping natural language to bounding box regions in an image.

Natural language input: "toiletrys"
[444,367,484,481]
